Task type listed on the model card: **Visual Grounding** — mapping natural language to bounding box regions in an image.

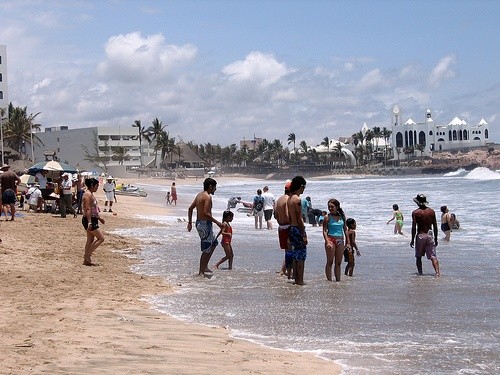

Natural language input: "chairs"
[67,190,83,214]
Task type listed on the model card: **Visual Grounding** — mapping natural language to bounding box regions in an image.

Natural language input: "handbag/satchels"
[25,194,31,199]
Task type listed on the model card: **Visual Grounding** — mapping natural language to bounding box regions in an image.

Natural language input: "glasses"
[300,184,306,188]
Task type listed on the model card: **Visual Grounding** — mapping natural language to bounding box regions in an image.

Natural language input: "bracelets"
[88,223,92,224]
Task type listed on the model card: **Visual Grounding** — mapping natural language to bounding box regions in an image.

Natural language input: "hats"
[414,193,429,206]
[0,163,11,171]
[34,182,40,187]
[62,173,69,177]
[285,181,292,190]
[107,176,114,180]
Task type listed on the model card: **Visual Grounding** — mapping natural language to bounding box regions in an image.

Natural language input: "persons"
[250,189,263,229]
[410,194,440,277]
[0,170,88,217]
[344,218,361,276]
[170,182,177,205]
[0,164,21,221]
[60,172,77,218]
[226,196,242,211]
[82,178,105,266]
[187,177,224,276]
[440,206,452,241]
[387,203,405,236]
[300,196,327,226]
[322,199,351,282]
[450,213,460,230]
[274,182,290,275]
[103,175,117,212]
[165,192,172,205]
[261,186,276,229]
[215,211,234,270]
[287,176,308,285]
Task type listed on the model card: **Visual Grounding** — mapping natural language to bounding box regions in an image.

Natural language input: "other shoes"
[109,209,112,212]
[103,209,107,212]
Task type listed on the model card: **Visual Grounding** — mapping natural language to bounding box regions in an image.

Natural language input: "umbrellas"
[24,159,78,176]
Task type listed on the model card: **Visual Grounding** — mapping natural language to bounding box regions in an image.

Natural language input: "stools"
[27,204,38,213]
